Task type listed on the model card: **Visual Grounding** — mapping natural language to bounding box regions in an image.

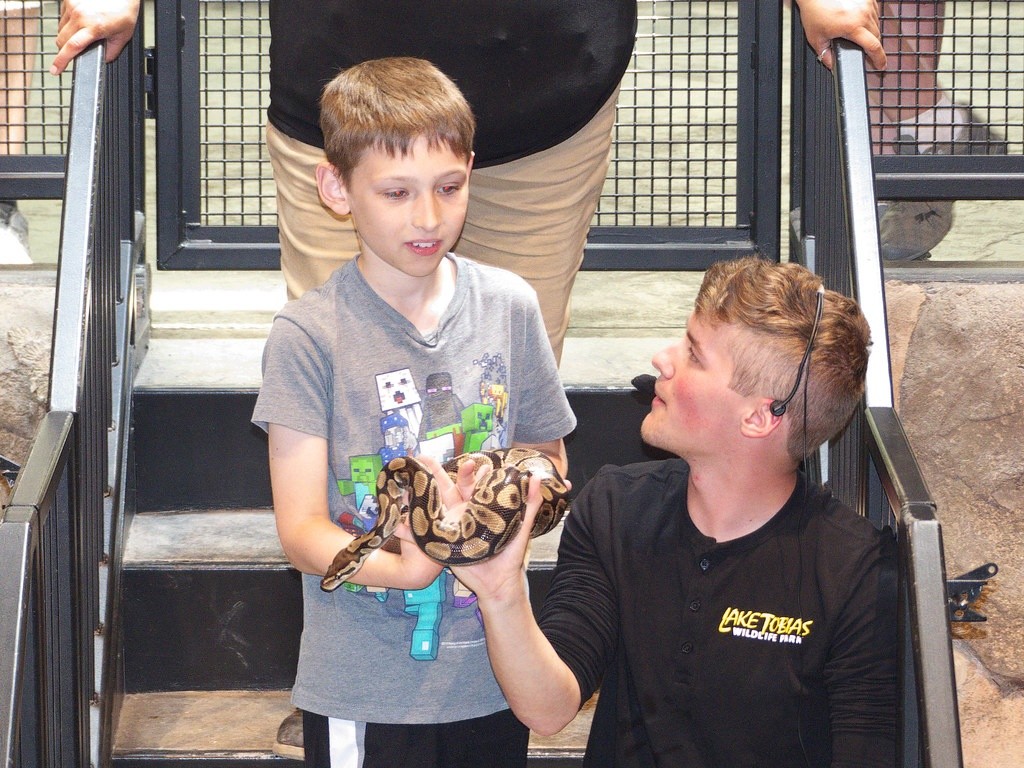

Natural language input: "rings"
[818,46,832,62]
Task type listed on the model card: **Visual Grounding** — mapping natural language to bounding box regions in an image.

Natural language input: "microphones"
[631,374,658,393]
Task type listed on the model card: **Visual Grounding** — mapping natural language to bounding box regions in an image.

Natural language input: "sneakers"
[879,102,1004,261]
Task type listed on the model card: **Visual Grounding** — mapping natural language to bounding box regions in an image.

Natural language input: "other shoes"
[273,708,306,760]
[0,212,33,264]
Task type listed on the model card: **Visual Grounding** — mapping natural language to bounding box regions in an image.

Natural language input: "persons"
[251,56,578,768]
[47,0,886,758]
[393,255,907,768]
[861,0,1008,265]
